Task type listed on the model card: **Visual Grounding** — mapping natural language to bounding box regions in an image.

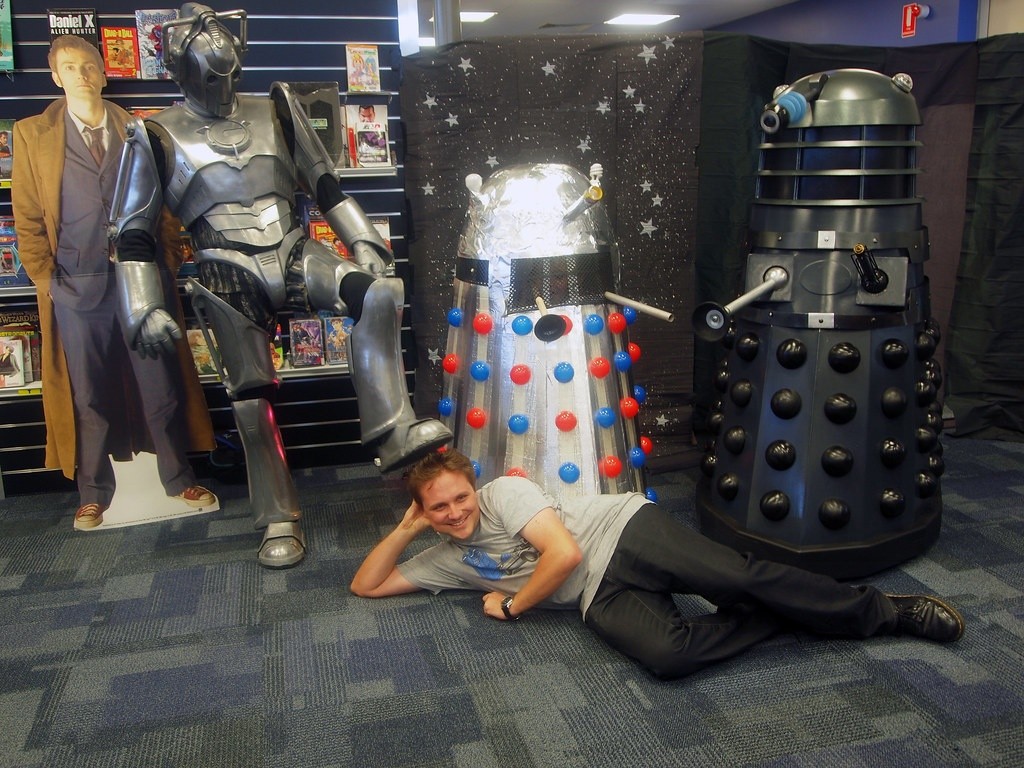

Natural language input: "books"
[270,316,354,370]
[0,215,30,287]
[346,44,381,92]
[0,0,14,70]
[0,119,16,179]
[186,329,222,373]
[345,104,392,169]
[45,8,180,79]
[0,323,40,388]
[309,216,395,276]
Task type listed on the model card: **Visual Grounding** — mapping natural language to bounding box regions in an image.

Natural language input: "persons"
[11,34,216,530]
[0,131,11,157]
[351,450,966,681]
[106,3,454,570]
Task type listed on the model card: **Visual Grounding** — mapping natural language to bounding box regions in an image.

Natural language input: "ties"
[82,127,106,168]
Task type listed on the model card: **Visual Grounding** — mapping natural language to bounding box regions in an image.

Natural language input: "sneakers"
[169,483,215,507]
[74,502,109,528]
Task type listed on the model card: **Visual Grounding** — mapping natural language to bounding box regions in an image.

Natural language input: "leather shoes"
[884,592,965,642]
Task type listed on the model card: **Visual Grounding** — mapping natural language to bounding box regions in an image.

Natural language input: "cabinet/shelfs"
[0,92,411,499]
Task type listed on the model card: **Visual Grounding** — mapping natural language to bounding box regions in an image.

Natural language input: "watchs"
[501,596,520,621]
